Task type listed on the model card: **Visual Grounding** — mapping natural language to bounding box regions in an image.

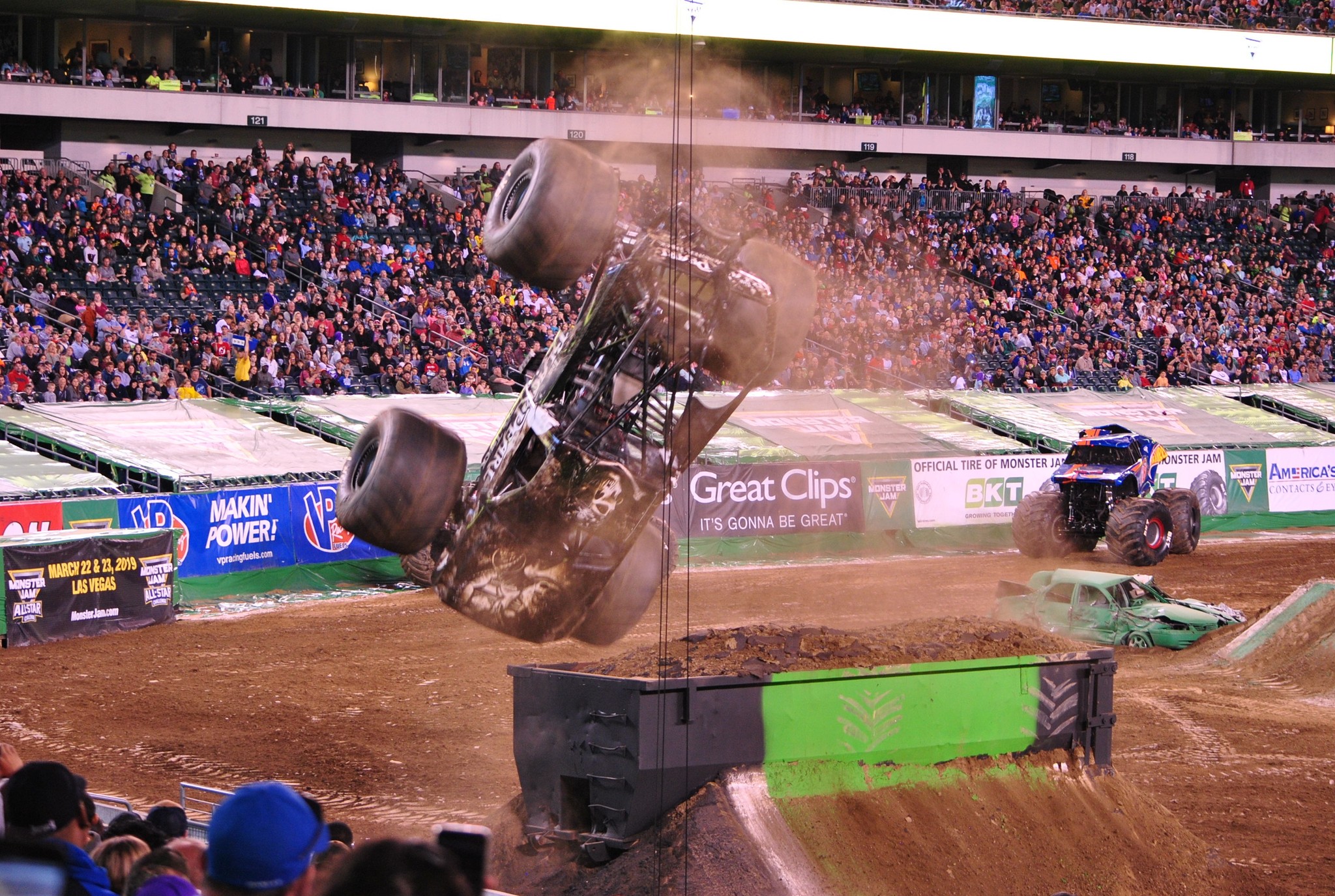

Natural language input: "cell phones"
[435,822,492,895]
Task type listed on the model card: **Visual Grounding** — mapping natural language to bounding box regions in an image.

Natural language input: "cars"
[1075,574,1248,626]
[997,569,1220,651]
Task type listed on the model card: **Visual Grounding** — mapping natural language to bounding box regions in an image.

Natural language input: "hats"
[203,775,331,892]
[0,761,93,842]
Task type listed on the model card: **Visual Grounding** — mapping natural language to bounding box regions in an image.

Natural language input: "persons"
[0,1,1332,414]
[1,737,501,896]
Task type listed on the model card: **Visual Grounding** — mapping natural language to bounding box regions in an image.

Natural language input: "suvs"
[1010,423,1201,567]
[334,136,818,647]
